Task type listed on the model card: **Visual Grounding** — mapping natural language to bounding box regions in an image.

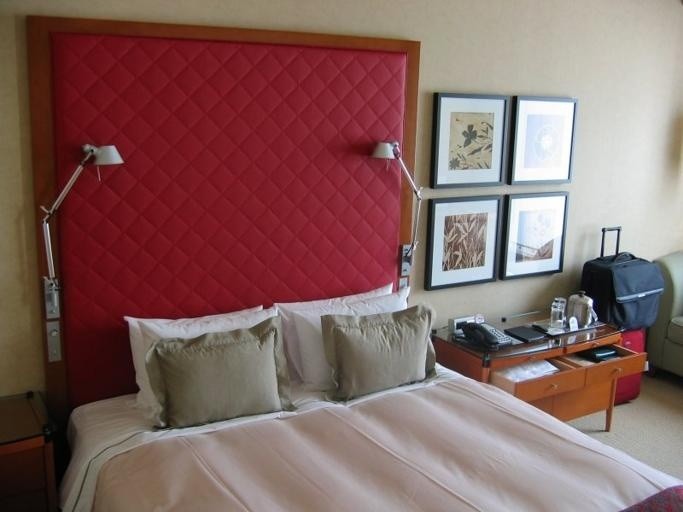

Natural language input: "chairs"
[647,250,683,379]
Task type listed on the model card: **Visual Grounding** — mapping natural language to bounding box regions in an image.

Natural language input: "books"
[503,325,545,345]
[560,346,621,369]
[501,360,561,382]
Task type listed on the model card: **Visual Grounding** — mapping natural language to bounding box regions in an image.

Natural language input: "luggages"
[601,226,645,405]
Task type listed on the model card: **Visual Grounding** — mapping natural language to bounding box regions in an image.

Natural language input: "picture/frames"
[424,90,580,291]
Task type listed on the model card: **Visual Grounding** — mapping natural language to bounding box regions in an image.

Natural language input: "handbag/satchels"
[582,253,664,331]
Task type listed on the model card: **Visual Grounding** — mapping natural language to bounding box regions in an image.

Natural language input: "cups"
[550,297,565,329]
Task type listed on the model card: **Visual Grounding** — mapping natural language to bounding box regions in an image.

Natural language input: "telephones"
[462,322,513,351]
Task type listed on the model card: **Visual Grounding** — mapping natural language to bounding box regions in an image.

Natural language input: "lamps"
[371,140,423,277]
[39,142,124,319]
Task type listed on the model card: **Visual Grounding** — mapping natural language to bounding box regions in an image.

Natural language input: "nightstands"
[0,390,59,512]
[431,310,649,433]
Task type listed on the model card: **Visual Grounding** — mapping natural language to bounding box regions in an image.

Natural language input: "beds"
[28,18,683,512]
